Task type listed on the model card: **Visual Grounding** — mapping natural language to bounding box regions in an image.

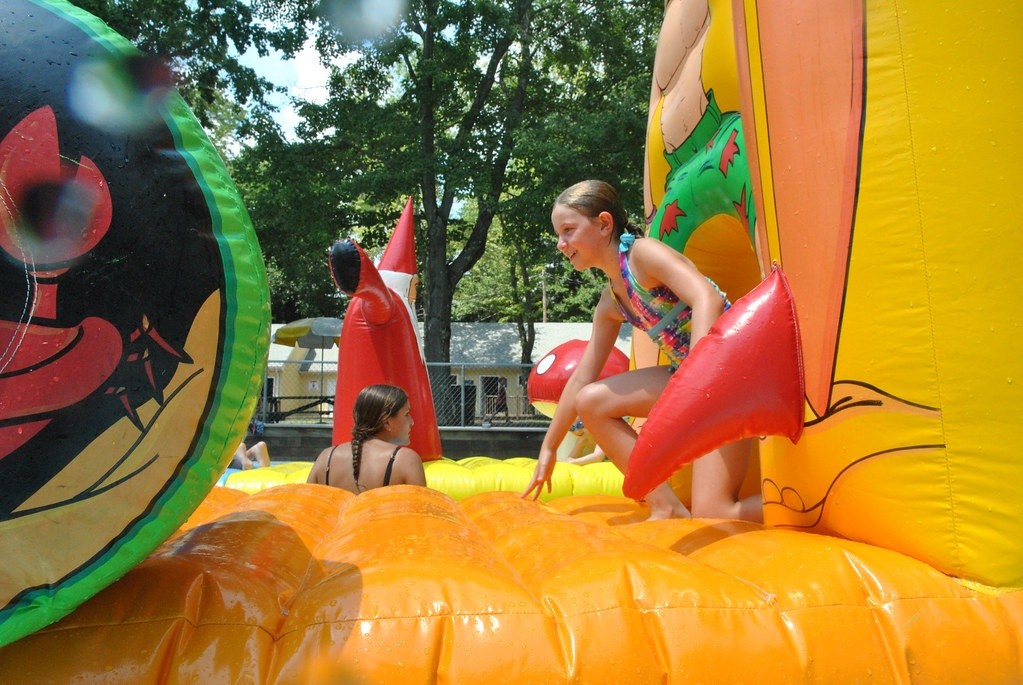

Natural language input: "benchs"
[266,411,334,415]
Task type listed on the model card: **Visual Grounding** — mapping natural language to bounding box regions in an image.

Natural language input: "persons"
[230,441,270,470]
[521,180,763,523]
[488,378,513,423]
[306,384,427,495]
[567,417,605,465]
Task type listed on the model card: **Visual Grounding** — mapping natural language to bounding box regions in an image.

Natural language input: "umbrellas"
[271,315,344,423]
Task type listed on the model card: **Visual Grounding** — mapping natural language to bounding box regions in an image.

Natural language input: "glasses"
[567,421,585,432]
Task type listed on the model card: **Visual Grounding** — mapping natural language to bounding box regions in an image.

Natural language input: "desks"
[269,396,335,423]
